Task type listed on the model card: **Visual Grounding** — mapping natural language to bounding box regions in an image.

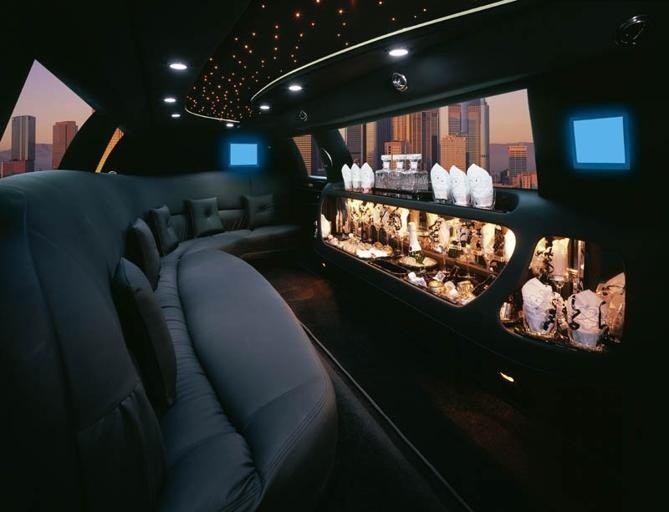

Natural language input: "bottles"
[431,220,505,273]
[341,211,406,253]
[471,274,496,297]
[407,221,425,264]
[441,265,459,288]
[374,153,429,200]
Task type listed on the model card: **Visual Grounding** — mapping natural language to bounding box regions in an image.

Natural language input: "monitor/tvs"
[568,110,631,169]
[230,143,258,166]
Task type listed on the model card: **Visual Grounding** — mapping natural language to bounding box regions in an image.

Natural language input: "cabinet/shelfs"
[319,181,640,386]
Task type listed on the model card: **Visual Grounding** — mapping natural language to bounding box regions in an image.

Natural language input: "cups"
[429,280,446,294]
[343,171,373,195]
[565,304,606,349]
[430,172,497,211]
[522,295,558,336]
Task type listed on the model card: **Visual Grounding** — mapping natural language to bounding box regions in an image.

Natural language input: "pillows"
[111,196,277,409]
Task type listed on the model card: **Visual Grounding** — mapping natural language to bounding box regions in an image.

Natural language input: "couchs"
[2,164,474,511]
